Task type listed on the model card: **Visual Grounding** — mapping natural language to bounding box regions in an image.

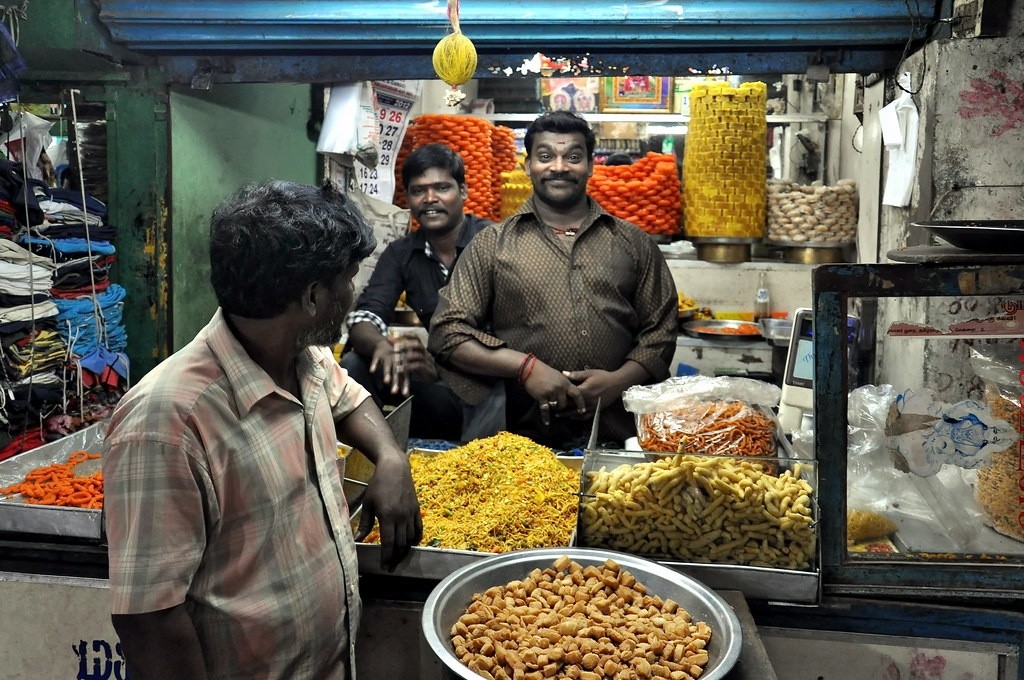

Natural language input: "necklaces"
[550,226,578,235]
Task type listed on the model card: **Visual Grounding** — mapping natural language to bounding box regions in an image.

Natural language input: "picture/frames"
[599,76,673,114]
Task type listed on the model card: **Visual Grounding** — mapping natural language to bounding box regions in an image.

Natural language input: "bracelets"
[517,352,536,383]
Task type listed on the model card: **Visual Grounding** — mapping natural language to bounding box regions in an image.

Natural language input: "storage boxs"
[811,260,1024,611]
[0,418,106,538]
[669,335,773,379]
[573,397,818,571]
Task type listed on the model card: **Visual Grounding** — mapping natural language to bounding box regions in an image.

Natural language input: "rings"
[540,404,549,409]
[549,401,557,406]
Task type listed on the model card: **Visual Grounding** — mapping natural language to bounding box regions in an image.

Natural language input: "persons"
[338,146,499,440]
[104,182,423,680]
[427,110,678,455]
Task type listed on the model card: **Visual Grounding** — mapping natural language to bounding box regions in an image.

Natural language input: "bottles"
[754,271,770,323]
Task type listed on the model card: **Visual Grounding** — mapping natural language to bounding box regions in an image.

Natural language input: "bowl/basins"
[421,547,742,680]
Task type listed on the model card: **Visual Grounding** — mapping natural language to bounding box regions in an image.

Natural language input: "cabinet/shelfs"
[475,110,829,271]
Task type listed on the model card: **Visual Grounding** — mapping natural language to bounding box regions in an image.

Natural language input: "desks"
[716,589,777,680]
